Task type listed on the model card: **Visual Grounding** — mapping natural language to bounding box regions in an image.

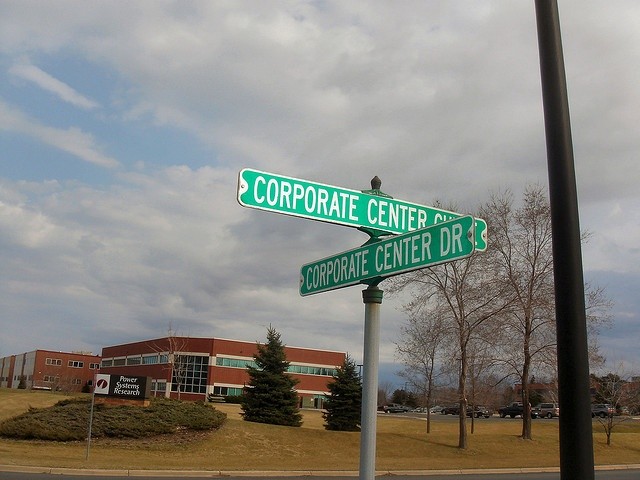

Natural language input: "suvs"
[592,403,617,418]
[466,405,491,418]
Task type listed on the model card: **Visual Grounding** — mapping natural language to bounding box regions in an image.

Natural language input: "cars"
[383,403,409,414]
[440,404,461,415]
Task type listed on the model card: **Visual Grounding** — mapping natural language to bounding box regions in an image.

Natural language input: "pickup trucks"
[498,401,541,419]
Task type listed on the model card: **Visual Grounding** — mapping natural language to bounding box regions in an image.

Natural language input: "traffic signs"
[299,214,474,296]
[237,168,488,253]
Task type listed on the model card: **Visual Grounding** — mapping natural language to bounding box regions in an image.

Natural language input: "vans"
[540,403,560,418]
